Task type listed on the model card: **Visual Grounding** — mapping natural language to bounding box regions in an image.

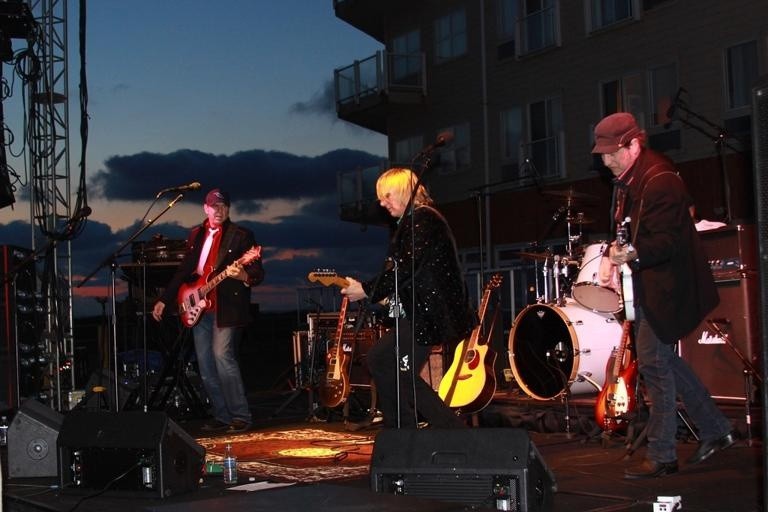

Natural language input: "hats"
[592,112,640,153]
[205,188,230,207]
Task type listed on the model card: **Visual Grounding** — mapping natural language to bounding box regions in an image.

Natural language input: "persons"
[589,110,746,486]
[149,187,268,435]
[338,167,478,430]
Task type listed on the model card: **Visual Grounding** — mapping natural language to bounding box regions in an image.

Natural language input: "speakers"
[54,409,207,497]
[372,426,557,512]
[678,275,760,406]
[4,395,58,480]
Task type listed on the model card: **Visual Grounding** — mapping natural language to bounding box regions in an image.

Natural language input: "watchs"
[626,245,639,263]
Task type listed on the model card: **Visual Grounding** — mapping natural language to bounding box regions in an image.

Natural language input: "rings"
[230,272,233,276]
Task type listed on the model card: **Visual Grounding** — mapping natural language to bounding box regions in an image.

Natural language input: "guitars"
[319,294,350,409]
[594,320,638,432]
[615,216,638,320]
[177,245,263,328]
[307,268,351,288]
[437,272,504,415]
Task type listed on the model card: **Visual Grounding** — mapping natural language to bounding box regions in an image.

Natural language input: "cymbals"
[516,251,563,261]
[542,190,590,198]
[564,216,597,224]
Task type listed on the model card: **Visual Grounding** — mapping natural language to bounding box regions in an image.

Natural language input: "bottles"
[221,441,239,486]
[73,452,83,486]
[139,449,154,488]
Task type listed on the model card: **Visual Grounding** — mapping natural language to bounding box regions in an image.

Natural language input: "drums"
[571,240,624,314]
[507,302,632,402]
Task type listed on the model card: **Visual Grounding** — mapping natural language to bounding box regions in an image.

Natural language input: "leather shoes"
[200,419,249,433]
[624,461,678,480]
[686,430,738,464]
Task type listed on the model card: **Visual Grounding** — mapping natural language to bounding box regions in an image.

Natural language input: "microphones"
[412,132,453,161]
[708,316,731,326]
[60,206,92,226]
[156,181,203,200]
